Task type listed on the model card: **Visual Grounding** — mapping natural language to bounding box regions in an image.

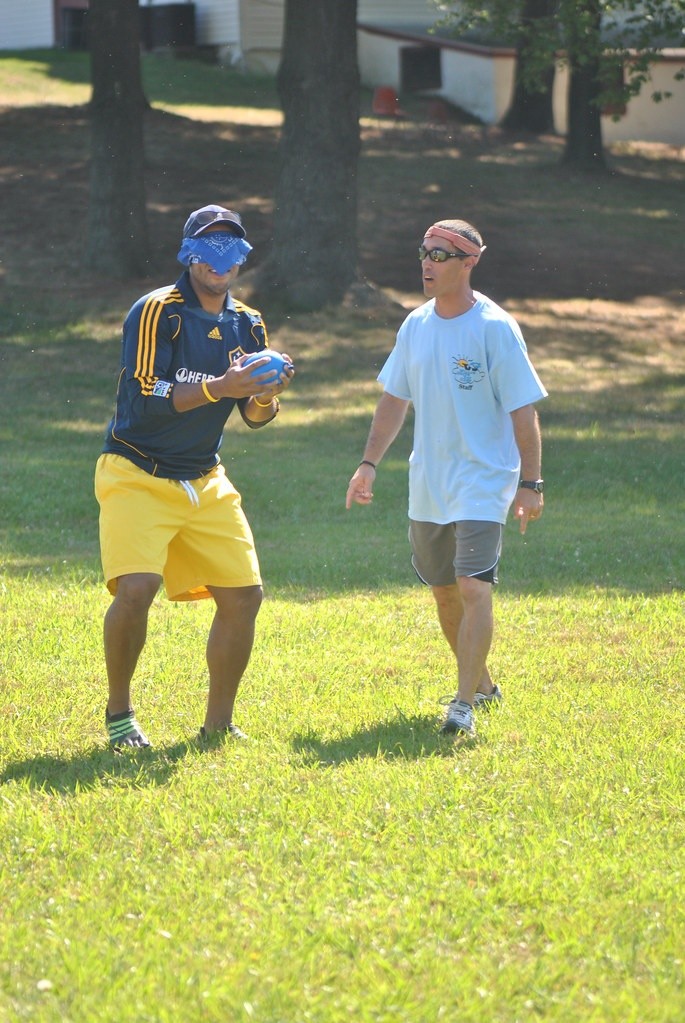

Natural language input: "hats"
[183,204,246,239]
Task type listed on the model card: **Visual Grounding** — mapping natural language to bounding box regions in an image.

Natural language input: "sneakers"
[438,700,477,742]
[193,723,258,748]
[470,683,506,710]
[105,704,154,754]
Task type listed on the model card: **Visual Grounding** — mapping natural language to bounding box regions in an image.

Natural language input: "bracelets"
[253,395,273,408]
[201,378,221,404]
[359,459,376,469]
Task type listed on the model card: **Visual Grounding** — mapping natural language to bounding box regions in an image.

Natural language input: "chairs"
[372,88,410,120]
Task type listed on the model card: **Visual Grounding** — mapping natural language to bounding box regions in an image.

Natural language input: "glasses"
[183,210,242,237]
[417,245,479,262]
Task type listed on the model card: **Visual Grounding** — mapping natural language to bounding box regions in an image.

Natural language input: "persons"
[92,202,296,764]
[343,219,548,737]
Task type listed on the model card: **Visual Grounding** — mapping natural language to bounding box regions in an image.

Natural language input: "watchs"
[518,479,545,495]
